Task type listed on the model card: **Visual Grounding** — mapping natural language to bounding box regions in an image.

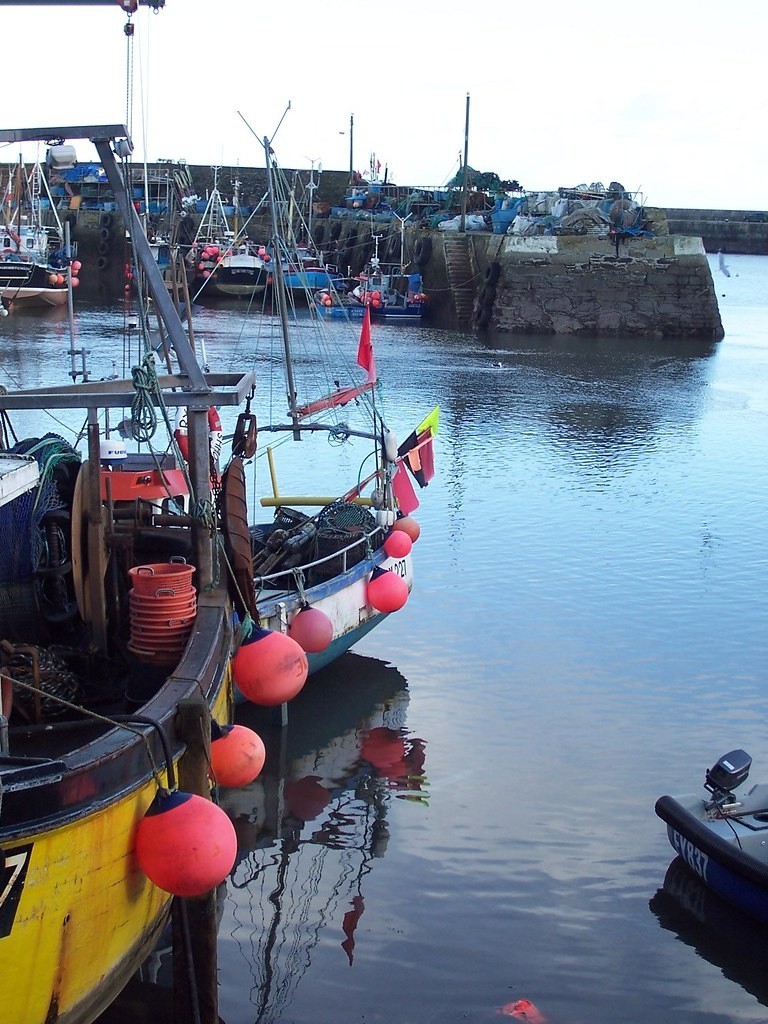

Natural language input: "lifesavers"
[30,508,80,624]
[478,286,497,305]
[100,214,112,227]
[174,405,223,465]
[366,194,378,208]
[314,223,403,268]
[97,241,109,255]
[98,228,110,240]
[413,236,432,265]
[95,256,109,270]
[53,245,77,267]
[64,215,76,226]
[483,261,500,285]
[473,305,491,326]
[0,249,15,262]
[298,218,309,238]
[610,199,637,226]
[242,185,269,216]
[217,173,239,199]
[469,192,485,204]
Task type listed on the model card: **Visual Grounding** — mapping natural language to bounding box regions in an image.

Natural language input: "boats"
[653,748,768,932]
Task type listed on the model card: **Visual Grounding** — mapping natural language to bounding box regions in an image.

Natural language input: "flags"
[390,406,442,516]
[358,303,377,383]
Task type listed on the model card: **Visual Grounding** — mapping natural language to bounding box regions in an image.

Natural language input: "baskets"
[128,556,196,676]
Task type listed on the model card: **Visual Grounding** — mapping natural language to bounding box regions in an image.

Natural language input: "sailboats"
[0,84,489,1024]
